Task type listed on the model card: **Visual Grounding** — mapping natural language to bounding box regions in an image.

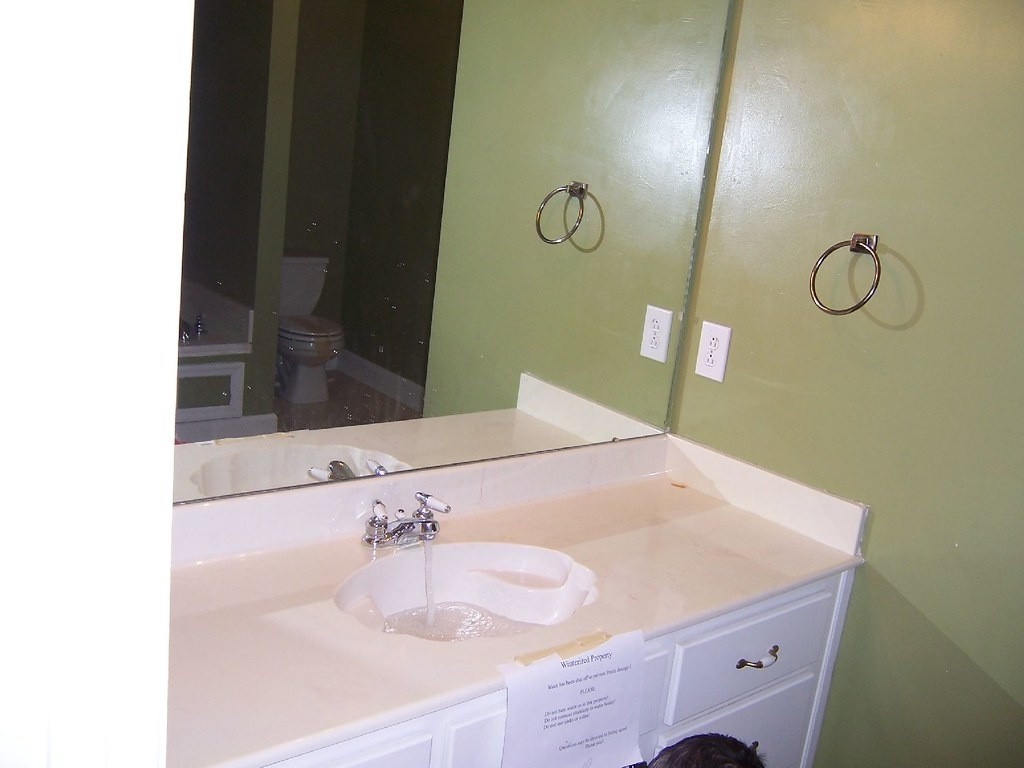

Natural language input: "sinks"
[333,540,602,644]
[189,442,414,499]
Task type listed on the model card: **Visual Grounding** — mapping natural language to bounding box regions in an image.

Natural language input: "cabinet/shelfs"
[262,570,857,768]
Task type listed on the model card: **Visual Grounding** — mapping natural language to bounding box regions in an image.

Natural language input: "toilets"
[277,255,346,404]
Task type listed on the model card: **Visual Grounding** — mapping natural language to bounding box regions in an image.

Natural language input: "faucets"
[329,461,357,480]
[388,509,440,546]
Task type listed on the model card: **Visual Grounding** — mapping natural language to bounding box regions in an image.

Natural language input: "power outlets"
[640,304,673,364]
[694,320,732,385]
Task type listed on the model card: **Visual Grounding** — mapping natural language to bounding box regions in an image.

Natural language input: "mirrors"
[173,0,731,506]
